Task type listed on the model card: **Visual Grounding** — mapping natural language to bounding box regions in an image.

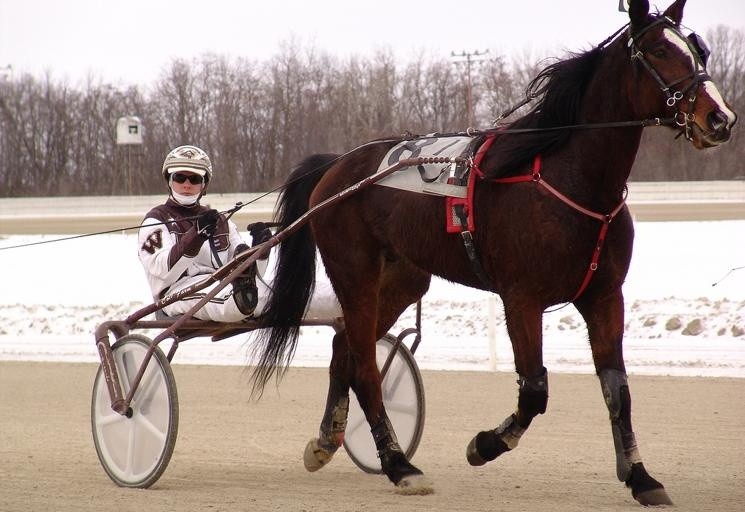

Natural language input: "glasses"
[170,173,203,185]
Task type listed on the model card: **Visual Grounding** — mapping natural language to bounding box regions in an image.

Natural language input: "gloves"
[196,209,218,239]
[248,222,272,260]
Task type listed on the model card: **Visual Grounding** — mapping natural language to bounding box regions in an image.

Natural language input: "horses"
[246,0,738,507]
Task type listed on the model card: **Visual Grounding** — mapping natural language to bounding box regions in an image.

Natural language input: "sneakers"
[232,243,259,315]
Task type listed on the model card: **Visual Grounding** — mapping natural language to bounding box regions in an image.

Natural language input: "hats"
[166,167,207,177]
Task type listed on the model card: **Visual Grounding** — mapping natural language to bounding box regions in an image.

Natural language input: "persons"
[138,145,344,324]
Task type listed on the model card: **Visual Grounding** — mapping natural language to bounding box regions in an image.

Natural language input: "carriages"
[91,2,737,507]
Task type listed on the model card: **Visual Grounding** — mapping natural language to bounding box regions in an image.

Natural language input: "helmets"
[162,145,212,183]
